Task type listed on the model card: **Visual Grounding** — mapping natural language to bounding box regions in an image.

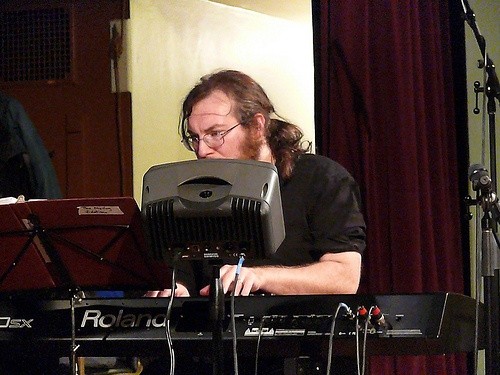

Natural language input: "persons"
[142,70,366,298]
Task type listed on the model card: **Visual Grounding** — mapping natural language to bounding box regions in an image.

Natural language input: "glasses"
[180,122,243,153]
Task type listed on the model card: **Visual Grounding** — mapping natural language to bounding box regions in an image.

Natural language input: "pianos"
[0,292,489,354]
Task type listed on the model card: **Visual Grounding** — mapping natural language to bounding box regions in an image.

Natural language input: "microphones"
[467,163,500,231]
